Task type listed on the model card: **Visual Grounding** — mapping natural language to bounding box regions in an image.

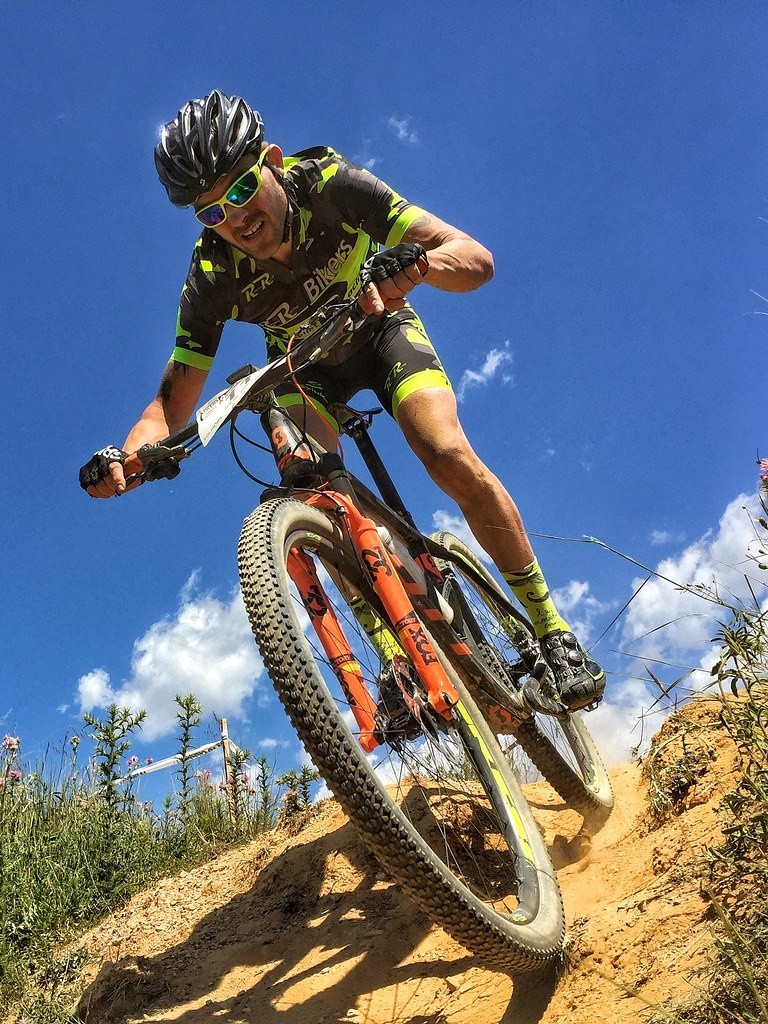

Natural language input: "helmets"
[154,89,265,209]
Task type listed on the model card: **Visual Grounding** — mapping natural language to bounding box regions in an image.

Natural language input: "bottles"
[375,527,454,625]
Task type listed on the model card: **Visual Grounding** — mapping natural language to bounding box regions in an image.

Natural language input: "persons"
[79,91,604,741]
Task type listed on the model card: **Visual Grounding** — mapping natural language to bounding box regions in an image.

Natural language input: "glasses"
[194,147,269,228]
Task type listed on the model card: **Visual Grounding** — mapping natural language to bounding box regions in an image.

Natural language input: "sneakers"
[376,657,422,719]
[541,630,606,709]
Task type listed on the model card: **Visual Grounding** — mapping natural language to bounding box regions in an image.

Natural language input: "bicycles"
[87,255,615,979]
